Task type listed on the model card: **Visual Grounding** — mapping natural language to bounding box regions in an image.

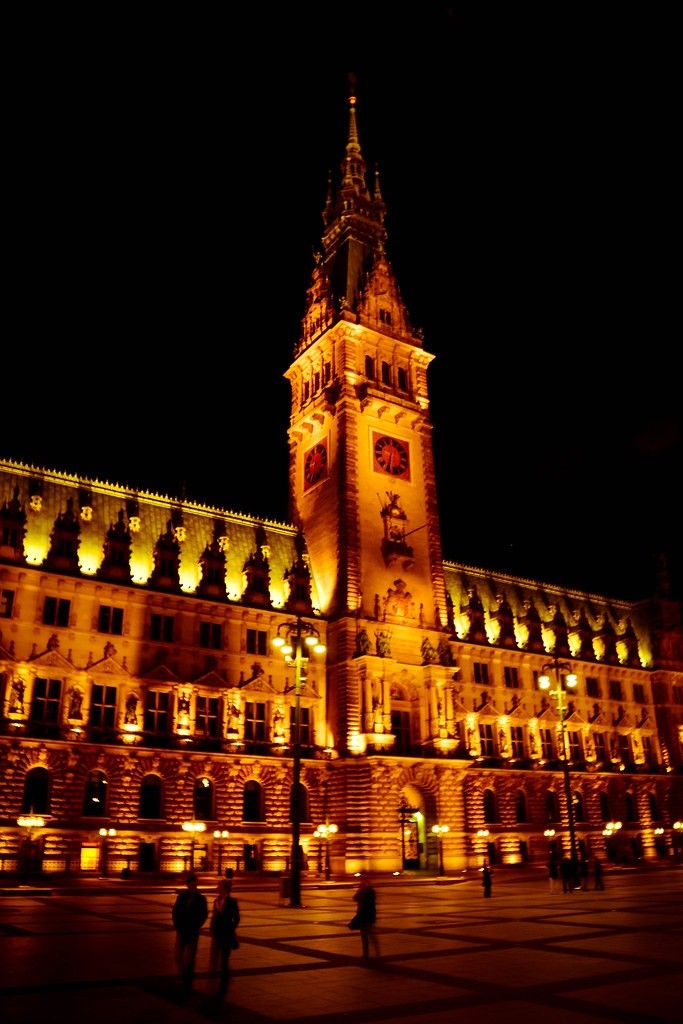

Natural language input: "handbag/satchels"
[230,933,239,950]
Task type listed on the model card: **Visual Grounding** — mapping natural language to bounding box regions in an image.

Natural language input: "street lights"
[270,607,329,908]
[536,645,583,887]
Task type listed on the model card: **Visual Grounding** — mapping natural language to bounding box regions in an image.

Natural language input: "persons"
[209,879,240,980]
[353,878,381,960]
[560,858,574,893]
[172,876,208,980]
[578,858,589,892]
[547,854,558,894]
[481,867,492,897]
[592,858,605,890]
